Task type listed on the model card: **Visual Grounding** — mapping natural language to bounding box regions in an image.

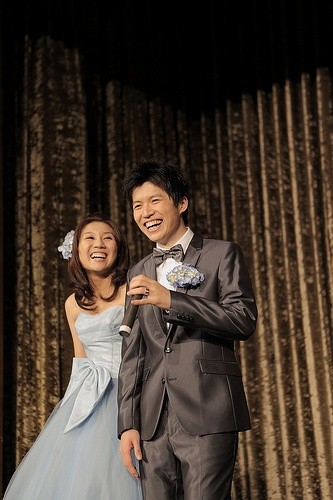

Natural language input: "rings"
[144,287,149,296]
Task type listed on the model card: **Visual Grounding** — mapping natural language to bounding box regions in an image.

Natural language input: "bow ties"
[153,244,184,267]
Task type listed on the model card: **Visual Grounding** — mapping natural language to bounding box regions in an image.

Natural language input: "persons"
[116,157,259,500]
[1,216,144,500]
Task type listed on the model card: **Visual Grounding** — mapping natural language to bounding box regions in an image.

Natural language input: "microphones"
[119,294,143,337]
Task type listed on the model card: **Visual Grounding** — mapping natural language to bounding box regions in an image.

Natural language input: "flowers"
[58,230,75,261]
[166,264,205,287]
